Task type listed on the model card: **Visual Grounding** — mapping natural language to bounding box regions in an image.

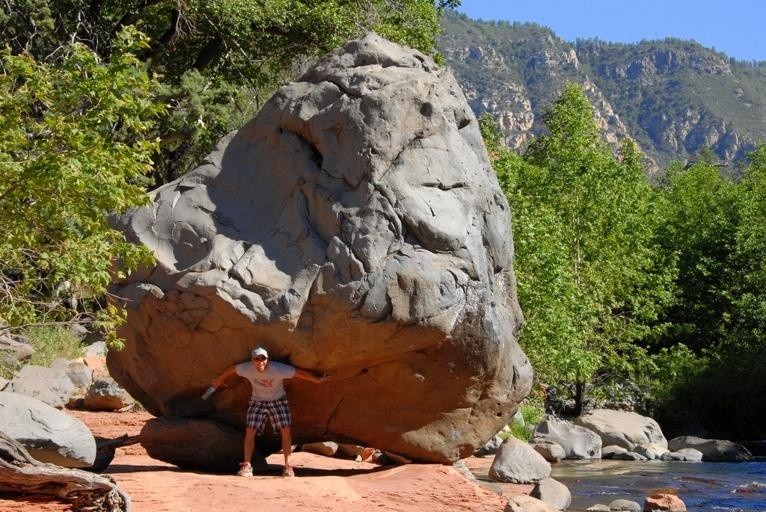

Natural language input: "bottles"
[201,386,215,401]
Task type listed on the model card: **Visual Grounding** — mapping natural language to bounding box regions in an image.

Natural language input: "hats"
[251,344,269,358]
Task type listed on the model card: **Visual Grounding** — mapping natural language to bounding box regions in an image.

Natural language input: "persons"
[210,344,332,477]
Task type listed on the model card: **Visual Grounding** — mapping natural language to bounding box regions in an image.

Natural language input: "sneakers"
[282,464,294,476]
[237,462,253,477]
